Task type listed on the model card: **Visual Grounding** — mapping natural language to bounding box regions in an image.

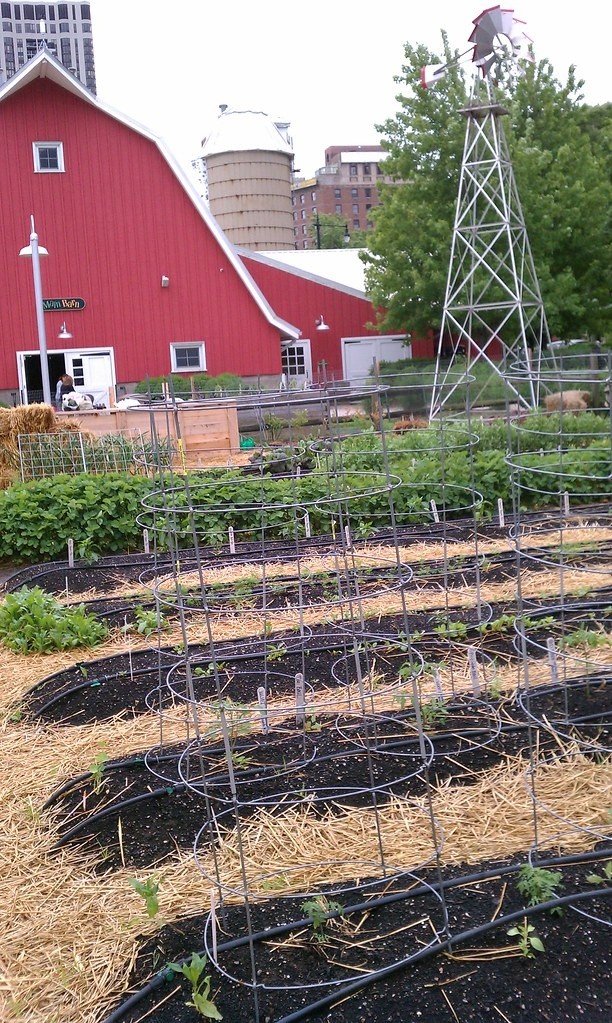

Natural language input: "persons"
[60,376,75,402]
[55,373,68,410]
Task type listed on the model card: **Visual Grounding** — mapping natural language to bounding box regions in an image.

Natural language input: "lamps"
[58,321,72,339]
[315,314,330,331]
[162,275,169,288]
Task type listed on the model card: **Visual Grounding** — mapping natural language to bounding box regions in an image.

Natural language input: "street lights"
[19,215,51,408]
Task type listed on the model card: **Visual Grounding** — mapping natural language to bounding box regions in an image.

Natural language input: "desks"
[56,407,120,418]
